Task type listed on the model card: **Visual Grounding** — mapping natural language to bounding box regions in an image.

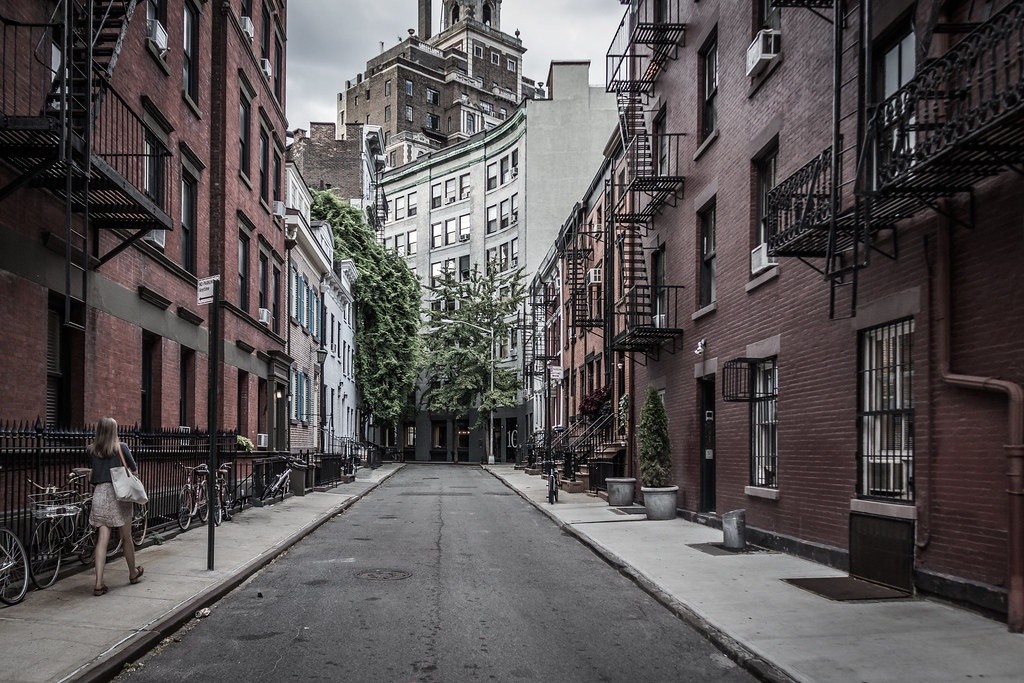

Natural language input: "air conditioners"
[258,308,271,325]
[511,215,517,223]
[241,17,255,43]
[257,434,269,448]
[510,167,518,175]
[746,29,782,77]
[750,242,779,277]
[459,234,470,241]
[147,18,168,50]
[586,268,603,286]
[511,260,518,267]
[273,201,286,219]
[593,224,602,237]
[444,197,453,205]
[179,426,191,446]
[461,192,469,200]
[261,59,272,77]
[143,229,166,252]
[652,314,666,328]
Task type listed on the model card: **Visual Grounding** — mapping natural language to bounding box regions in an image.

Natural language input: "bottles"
[46,506,81,515]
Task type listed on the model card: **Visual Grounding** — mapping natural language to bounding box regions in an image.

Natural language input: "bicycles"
[24,472,150,592]
[546,458,565,505]
[174,461,210,530]
[259,456,312,501]
[0,527,29,607]
[205,462,233,527]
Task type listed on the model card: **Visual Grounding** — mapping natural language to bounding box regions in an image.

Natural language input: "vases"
[604,477,637,506]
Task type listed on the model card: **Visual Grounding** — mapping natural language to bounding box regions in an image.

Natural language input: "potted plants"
[636,385,680,520]
[236,435,254,454]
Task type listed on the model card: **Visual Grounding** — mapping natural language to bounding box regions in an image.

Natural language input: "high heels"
[94,584,108,596]
[129,566,144,583]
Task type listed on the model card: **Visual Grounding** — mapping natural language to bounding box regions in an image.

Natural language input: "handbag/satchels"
[110,442,148,505]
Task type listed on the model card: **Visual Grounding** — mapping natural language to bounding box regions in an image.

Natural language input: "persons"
[84,417,144,596]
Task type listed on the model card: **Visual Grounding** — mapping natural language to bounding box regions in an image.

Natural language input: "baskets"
[27,490,84,519]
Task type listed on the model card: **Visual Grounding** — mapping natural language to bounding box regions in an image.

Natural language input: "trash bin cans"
[723,510,745,550]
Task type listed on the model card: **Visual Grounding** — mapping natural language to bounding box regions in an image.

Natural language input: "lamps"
[286,394,292,402]
[340,382,343,386]
[275,389,283,399]
[316,349,328,364]
[617,363,624,369]
[694,338,706,355]
[343,394,348,398]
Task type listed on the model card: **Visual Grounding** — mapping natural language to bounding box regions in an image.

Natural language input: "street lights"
[440,318,495,465]
